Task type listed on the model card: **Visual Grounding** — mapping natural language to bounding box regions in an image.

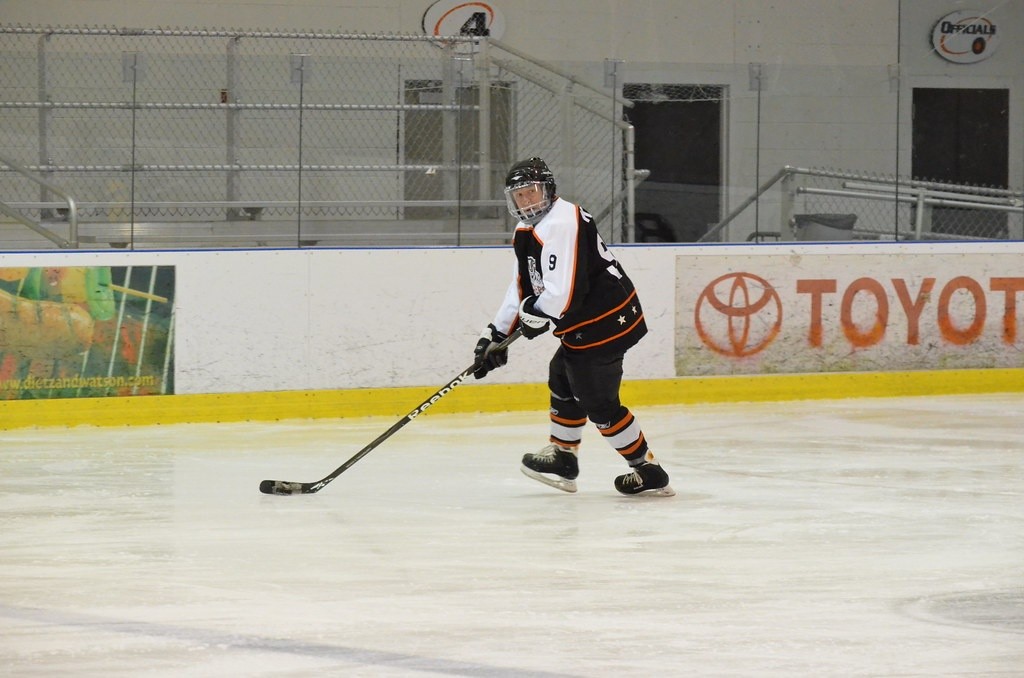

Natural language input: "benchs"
[0,216,515,251]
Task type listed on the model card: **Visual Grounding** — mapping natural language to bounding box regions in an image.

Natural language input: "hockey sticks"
[256,325,526,496]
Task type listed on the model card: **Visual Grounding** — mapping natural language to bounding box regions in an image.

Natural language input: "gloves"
[518,295,550,340]
[473,323,508,379]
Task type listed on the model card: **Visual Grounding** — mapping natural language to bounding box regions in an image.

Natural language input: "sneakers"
[520,442,579,493]
[614,459,675,497]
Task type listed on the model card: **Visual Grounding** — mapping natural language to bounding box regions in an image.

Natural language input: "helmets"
[506,157,557,225]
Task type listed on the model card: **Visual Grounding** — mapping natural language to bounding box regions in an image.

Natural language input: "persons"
[473,157,669,495]
